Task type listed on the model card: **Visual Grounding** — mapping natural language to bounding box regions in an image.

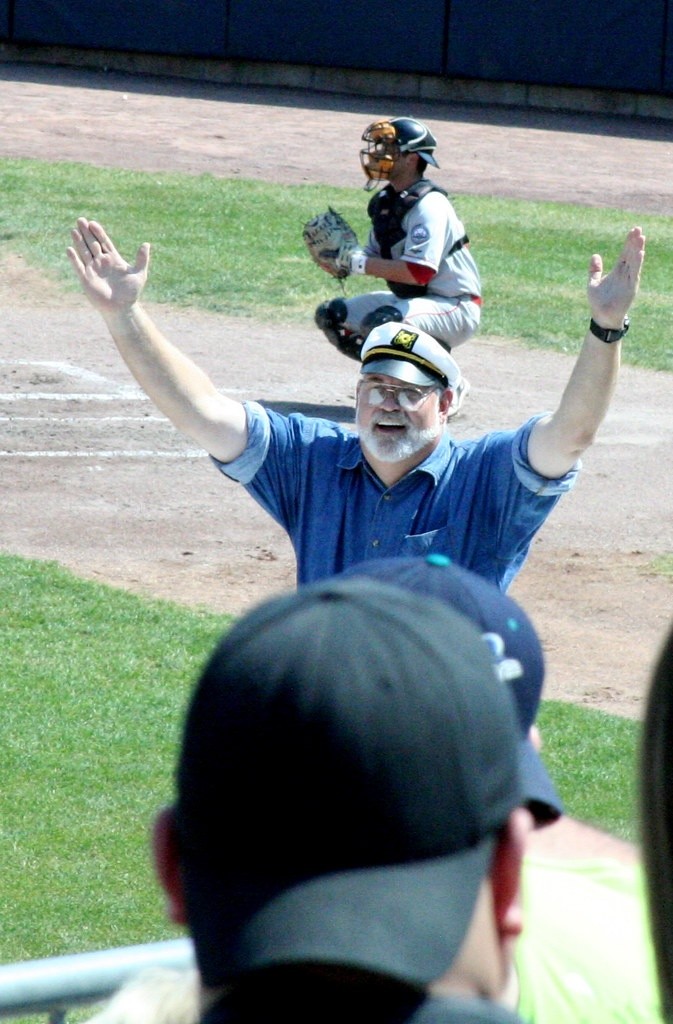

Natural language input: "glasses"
[360,379,444,413]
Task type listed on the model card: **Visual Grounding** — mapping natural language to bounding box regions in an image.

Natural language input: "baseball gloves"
[299,204,359,281]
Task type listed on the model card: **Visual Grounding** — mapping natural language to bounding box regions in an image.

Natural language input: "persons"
[302,116,481,424]
[82,555,673,1024]
[68,217,645,596]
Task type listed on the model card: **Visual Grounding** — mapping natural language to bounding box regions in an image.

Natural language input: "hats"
[338,550,563,827]
[361,322,463,395]
[173,573,525,990]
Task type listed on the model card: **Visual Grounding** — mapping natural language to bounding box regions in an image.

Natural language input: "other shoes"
[448,378,472,415]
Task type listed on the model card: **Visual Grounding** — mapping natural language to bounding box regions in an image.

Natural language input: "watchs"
[588,314,629,343]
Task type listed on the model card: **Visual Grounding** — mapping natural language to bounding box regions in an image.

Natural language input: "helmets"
[386,115,441,169]
